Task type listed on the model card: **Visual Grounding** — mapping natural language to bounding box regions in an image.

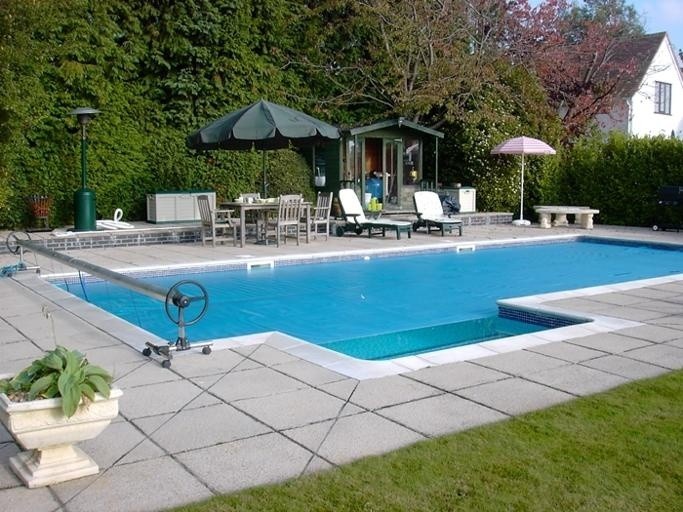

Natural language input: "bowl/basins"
[234,199,242,203]
[257,199,266,204]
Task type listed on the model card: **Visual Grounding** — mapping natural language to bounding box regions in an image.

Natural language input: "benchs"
[532,205,601,230]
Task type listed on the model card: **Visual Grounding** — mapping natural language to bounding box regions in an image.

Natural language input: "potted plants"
[0,346,125,490]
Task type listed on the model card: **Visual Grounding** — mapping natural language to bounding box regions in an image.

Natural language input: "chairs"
[337,188,464,241]
[195,191,335,249]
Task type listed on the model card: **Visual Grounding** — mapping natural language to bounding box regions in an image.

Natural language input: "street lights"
[66,107,102,232]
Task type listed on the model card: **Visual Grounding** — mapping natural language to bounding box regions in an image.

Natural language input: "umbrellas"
[490,135,557,219]
[183,96,341,244]
